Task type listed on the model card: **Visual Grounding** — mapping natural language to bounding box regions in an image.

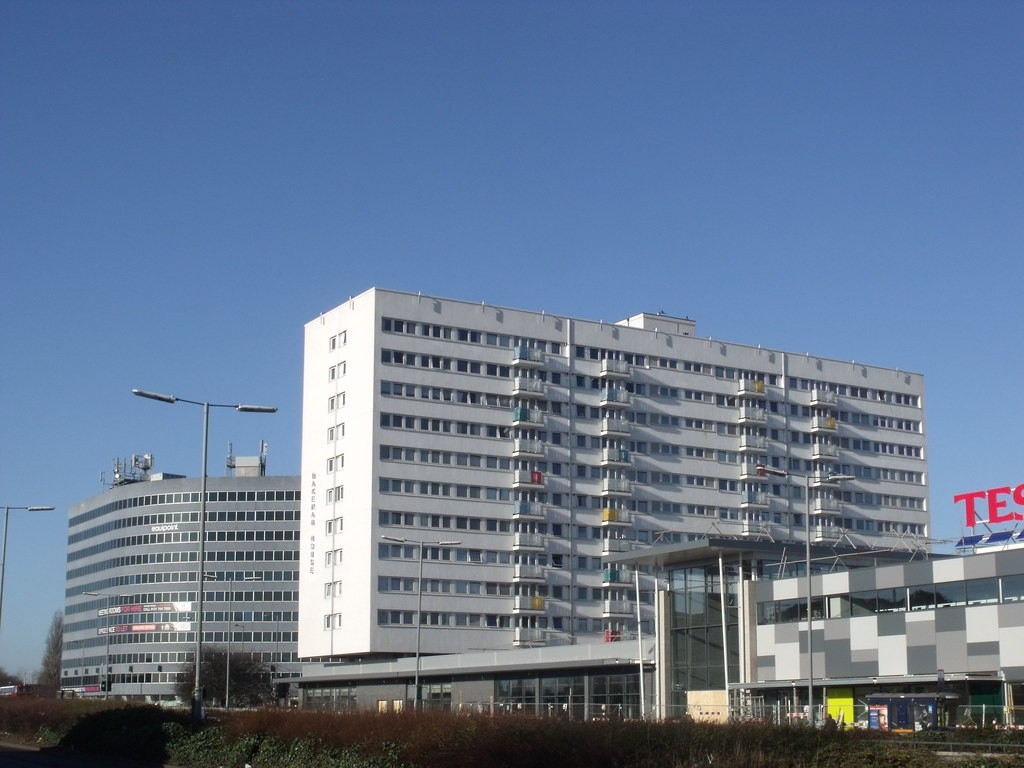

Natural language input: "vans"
[0,685,34,697]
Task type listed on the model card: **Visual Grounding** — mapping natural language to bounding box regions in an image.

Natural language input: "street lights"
[0,505,55,621]
[757,466,855,726]
[132,389,279,733]
[83,591,133,700]
[203,574,262,714]
[381,535,462,712]
